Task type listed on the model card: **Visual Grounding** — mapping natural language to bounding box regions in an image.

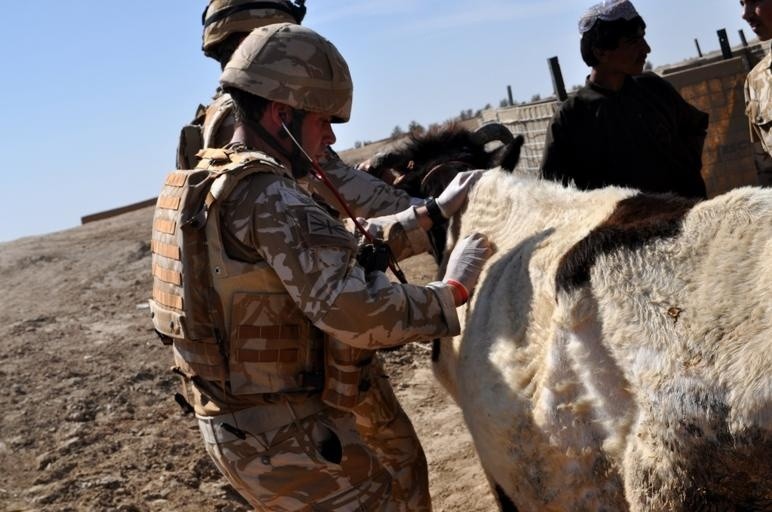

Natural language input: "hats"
[577,0,640,40]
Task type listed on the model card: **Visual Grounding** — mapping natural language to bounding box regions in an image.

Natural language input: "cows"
[354,119,772,512]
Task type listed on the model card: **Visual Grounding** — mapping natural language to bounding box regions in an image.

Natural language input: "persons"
[735,1,772,187]
[148,21,500,510]
[537,0,710,204]
[200,0,434,220]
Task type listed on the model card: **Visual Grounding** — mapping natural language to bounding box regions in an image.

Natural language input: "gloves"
[436,170,484,219]
[441,233,493,302]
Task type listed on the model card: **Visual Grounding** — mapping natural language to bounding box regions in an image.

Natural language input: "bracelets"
[424,196,446,225]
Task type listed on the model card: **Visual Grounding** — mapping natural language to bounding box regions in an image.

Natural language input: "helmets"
[221,22,352,123]
[202,1,306,54]
